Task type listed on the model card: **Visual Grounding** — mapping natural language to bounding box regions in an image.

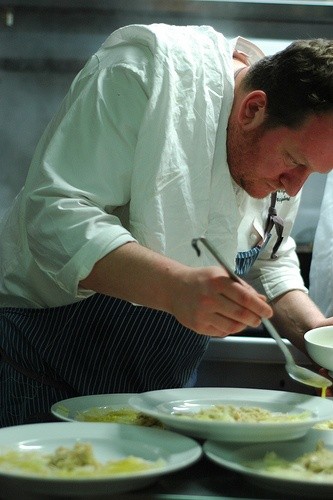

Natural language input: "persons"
[309,169,333,319]
[0,21,333,428]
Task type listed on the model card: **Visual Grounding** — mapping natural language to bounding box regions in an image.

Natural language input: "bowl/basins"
[303,325,333,378]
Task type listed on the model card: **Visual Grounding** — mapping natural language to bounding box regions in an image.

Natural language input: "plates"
[129,387,333,442]
[203,428,333,485]
[50,394,141,422]
[0,421,203,497]
